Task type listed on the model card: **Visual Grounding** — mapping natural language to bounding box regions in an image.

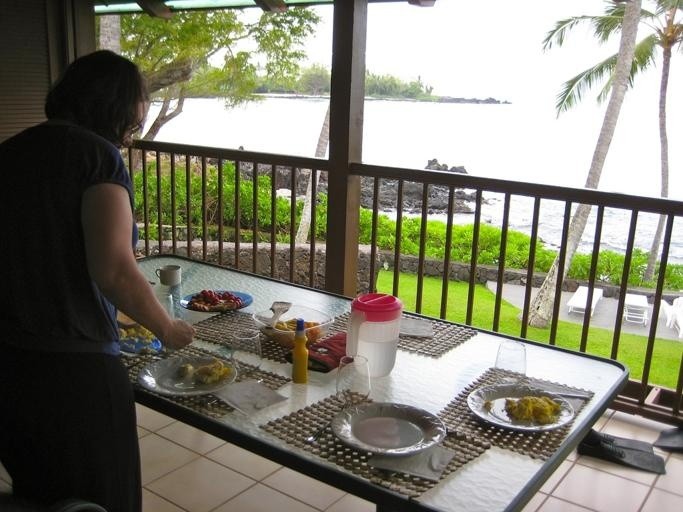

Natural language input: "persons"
[0,50,198,510]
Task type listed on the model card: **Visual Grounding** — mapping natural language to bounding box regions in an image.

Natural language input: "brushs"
[270,300,292,328]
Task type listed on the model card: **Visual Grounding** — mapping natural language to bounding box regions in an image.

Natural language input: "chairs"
[623,293,651,326]
[567,286,603,317]
[659,297,683,338]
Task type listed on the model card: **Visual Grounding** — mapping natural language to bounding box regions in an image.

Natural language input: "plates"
[178,290,252,313]
[136,356,237,396]
[467,384,575,432]
[333,402,447,457]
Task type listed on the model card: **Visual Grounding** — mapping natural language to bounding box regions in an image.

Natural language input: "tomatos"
[196,289,241,306]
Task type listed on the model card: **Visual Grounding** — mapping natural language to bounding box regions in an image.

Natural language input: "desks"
[120,253,629,512]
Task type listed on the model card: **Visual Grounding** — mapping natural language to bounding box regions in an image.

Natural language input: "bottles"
[291,317,310,383]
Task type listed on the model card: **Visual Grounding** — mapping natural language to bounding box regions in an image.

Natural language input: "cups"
[155,294,174,318]
[335,355,371,406]
[231,327,262,374]
[496,339,527,385]
[346,292,404,378]
[156,264,183,284]
